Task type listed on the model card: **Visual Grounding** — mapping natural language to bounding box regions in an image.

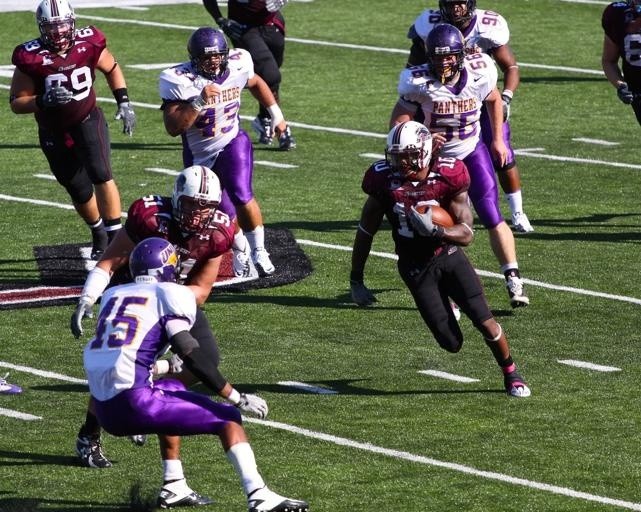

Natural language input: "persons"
[403,0,535,234]
[71,161,239,471]
[9,0,138,260]
[158,26,289,277]
[388,22,531,321]
[80,235,311,512]
[600,0,641,127]
[202,0,298,152]
[348,121,532,399]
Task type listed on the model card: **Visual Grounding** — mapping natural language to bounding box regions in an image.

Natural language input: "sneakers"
[251,247,275,276]
[0,375,23,394]
[248,484,310,512]
[76,434,112,468]
[511,213,534,232]
[504,373,530,399]
[253,115,274,145]
[276,124,297,149]
[231,234,257,278]
[157,478,215,507]
[506,275,530,307]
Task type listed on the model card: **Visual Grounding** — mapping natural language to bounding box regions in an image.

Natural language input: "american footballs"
[417,205,454,228]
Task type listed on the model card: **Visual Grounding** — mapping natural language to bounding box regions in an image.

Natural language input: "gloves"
[617,80,634,105]
[500,92,511,123]
[406,206,438,236]
[235,391,268,420]
[217,17,246,41]
[350,270,378,306]
[42,86,73,108]
[70,300,94,340]
[114,101,138,135]
[266,0,286,13]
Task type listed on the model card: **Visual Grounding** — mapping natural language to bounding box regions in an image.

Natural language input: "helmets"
[187,27,229,82]
[129,238,182,282]
[171,165,222,235]
[438,0,478,26]
[426,24,466,84]
[384,121,434,180]
[36,0,75,51]
